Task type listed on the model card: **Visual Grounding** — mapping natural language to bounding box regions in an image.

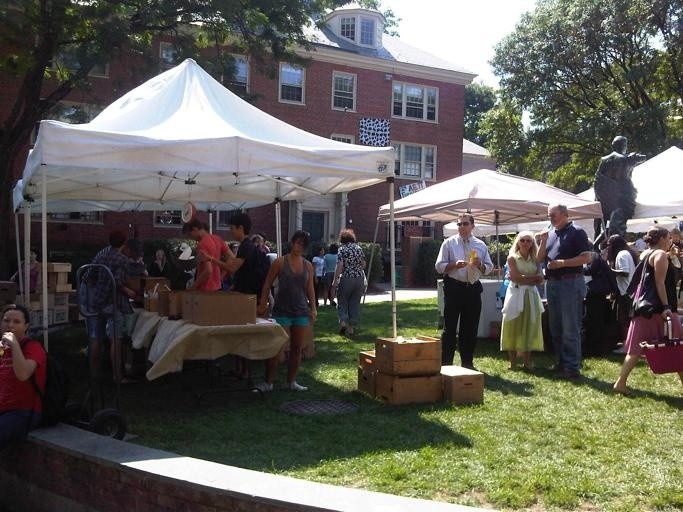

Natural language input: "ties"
[463,240,476,286]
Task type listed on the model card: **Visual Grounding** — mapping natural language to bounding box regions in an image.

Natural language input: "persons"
[182,219,234,291]
[256,230,317,391]
[312,244,339,308]
[435,212,494,370]
[2,305,47,447]
[200,214,261,379]
[501,229,683,354]
[500,232,544,367]
[124,232,272,310]
[80,230,138,383]
[594,135,647,238]
[332,231,364,334]
[537,206,591,377]
[614,226,683,393]
[22,247,40,293]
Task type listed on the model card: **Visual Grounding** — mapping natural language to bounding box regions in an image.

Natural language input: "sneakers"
[289,380,308,392]
[251,381,274,393]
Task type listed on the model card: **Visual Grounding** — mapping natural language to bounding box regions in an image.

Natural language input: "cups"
[470,249,478,263]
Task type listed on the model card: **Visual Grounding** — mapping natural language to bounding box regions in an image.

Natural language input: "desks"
[436,278,510,338]
[127,307,290,407]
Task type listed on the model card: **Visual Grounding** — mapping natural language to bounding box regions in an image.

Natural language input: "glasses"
[456,222,472,227]
[518,238,531,242]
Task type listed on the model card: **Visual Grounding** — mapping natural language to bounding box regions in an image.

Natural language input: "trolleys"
[50,258,133,444]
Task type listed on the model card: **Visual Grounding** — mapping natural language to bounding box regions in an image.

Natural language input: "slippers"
[612,388,636,399]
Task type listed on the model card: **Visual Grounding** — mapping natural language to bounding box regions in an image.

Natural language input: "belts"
[448,276,478,289]
[547,273,580,280]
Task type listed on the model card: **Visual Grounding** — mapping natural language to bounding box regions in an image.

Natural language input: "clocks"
[181,201,197,223]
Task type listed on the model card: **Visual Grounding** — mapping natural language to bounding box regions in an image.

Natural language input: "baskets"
[638,315,683,374]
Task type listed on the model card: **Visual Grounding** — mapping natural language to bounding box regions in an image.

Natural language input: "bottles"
[158,290,170,316]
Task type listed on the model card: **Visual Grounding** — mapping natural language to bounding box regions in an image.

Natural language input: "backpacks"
[19,336,71,429]
[240,240,272,294]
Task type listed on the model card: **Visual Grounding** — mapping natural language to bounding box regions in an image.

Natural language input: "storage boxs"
[0,280,17,301]
[359,338,484,407]
[12,261,78,328]
[142,276,258,326]
